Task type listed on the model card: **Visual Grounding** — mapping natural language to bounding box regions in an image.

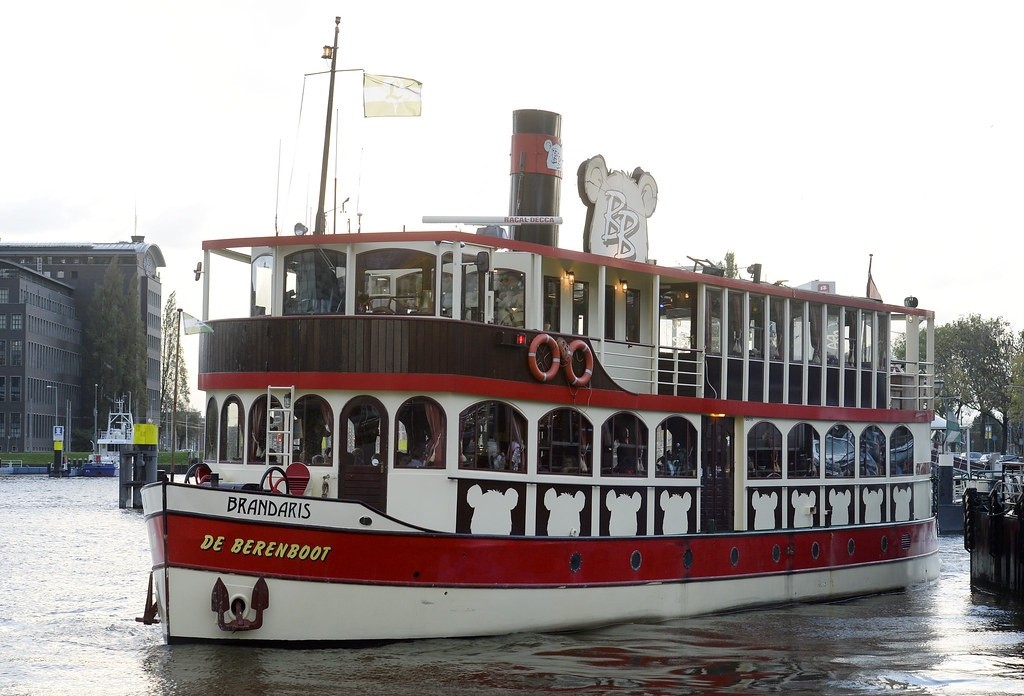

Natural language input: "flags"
[363,73,423,117]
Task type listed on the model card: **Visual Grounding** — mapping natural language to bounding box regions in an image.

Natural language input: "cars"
[959,452,1024,470]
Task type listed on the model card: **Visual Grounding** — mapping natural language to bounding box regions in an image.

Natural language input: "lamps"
[321,46,333,59]
[620,279,628,293]
[566,270,574,285]
[295,223,308,237]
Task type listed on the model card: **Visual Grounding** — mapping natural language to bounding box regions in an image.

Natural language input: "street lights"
[94,384,99,454]
[46,385,58,426]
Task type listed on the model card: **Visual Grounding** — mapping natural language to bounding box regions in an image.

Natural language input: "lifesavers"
[563,339,594,387]
[528,334,561,381]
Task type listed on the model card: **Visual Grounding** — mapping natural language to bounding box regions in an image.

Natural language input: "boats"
[138,14,945,643]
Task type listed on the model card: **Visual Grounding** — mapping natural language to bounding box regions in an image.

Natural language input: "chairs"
[271,463,310,495]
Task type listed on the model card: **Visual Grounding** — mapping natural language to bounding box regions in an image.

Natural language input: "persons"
[560,456,577,473]
[258,440,429,471]
[613,427,690,476]
[498,271,524,312]
